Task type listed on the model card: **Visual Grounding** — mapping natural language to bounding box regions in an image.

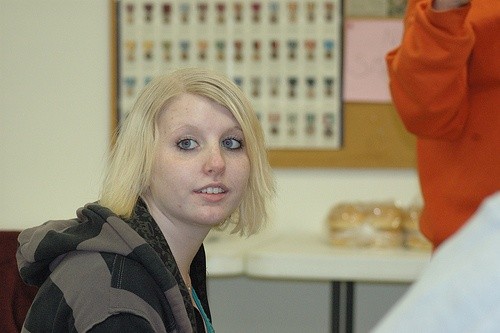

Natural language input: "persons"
[383,0,499,256]
[16,66,277,333]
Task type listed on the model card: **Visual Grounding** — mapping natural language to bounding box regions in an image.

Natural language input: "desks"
[202,229,432,333]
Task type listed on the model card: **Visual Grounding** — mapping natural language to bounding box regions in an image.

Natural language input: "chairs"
[1,231,43,333]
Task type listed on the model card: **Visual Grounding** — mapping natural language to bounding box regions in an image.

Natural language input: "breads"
[322,200,434,252]
